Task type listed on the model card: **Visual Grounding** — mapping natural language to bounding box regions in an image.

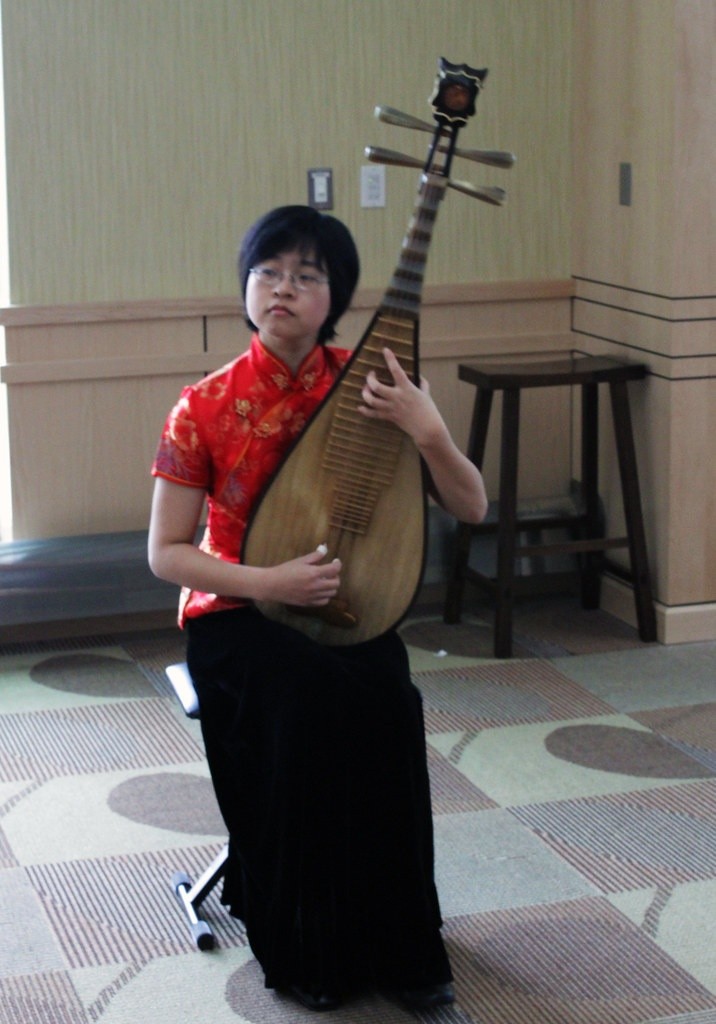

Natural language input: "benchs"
[1,521,214,646]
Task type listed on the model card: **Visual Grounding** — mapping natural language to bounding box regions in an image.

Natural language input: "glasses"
[248,266,330,293]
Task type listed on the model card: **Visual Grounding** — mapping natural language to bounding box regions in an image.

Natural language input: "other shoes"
[281,983,346,1011]
[384,982,456,1014]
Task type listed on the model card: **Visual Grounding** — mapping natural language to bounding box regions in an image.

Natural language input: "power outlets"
[359,164,387,209]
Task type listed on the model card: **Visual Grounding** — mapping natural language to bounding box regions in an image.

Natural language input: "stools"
[162,664,241,949]
[440,353,660,660]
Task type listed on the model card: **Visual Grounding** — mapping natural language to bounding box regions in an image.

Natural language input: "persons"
[146,204,490,1011]
